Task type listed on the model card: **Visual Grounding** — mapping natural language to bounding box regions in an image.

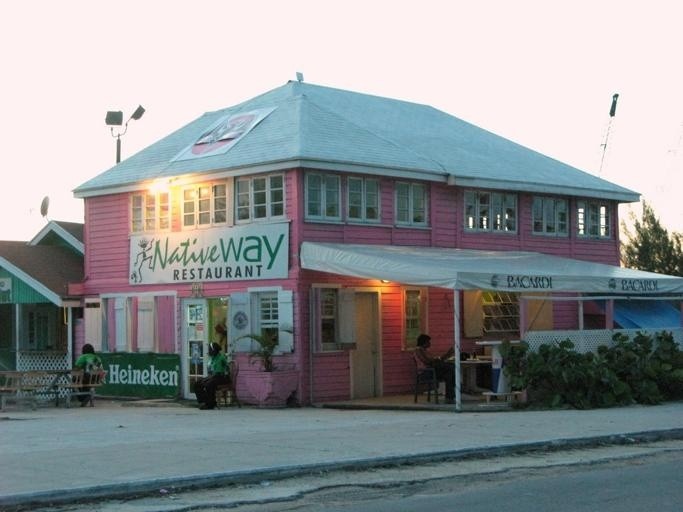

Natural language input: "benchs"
[0,385,57,409]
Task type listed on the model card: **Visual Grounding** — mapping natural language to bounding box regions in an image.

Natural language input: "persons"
[74,343,103,407]
[193,341,230,409]
[413,334,454,403]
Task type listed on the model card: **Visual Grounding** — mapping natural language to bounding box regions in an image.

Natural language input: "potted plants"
[500,340,544,403]
[230,322,297,407]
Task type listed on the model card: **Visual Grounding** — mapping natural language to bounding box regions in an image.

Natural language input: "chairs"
[409,352,450,403]
[56,369,101,406]
[214,359,239,408]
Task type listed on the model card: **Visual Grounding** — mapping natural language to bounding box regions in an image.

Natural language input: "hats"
[417,335,430,346]
[210,342,221,352]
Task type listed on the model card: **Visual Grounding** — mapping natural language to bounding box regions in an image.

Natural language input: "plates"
[467,359,479,362]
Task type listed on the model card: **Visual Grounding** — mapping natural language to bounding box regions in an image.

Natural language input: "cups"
[469,352,474,359]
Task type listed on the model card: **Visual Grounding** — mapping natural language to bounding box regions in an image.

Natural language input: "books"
[482,291,520,331]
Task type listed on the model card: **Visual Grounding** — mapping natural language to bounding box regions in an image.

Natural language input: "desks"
[443,355,492,401]
[0,369,68,385]
